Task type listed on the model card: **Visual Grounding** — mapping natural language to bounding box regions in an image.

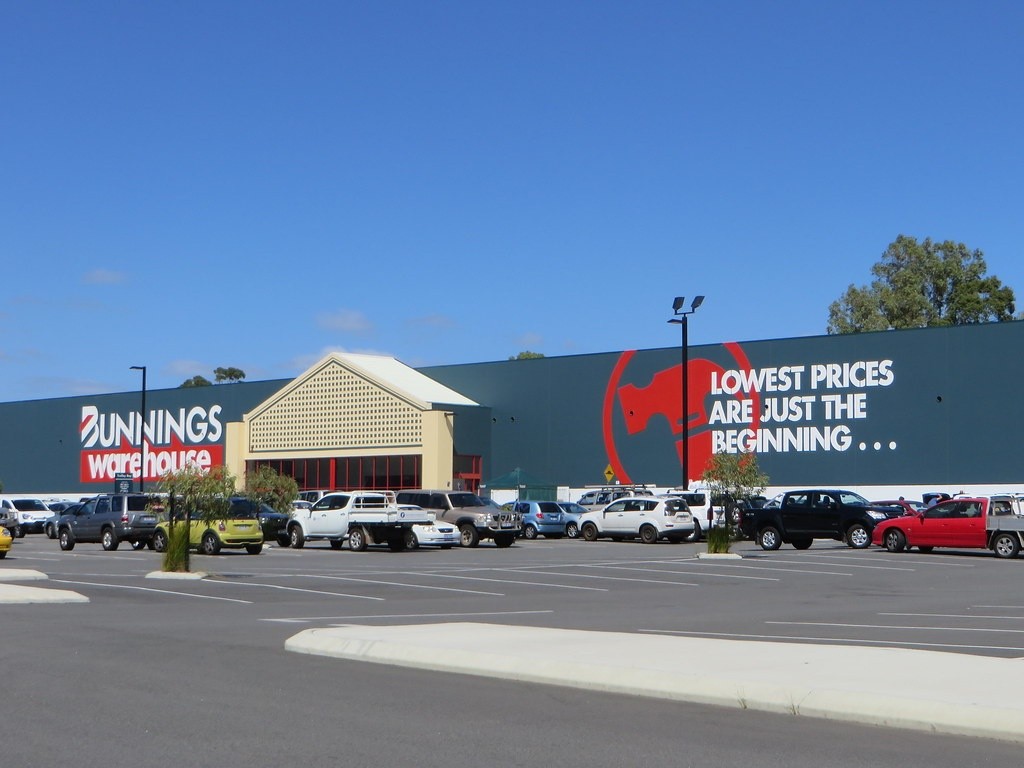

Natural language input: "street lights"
[131,364,149,492]
[667,294,705,489]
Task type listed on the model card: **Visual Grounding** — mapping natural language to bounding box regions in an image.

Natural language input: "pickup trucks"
[872,498,1024,558]
[286,490,436,550]
[740,489,902,548]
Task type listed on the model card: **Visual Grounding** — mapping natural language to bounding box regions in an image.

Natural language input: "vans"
[575,485,653,512]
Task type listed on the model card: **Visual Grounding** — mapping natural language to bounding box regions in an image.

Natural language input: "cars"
[576,496,696,545]
[290,488,344,510]
[555,501,590,538]
[870,497,929,516]
[153,496,263,555]
[257,499,291,546]
[1,497,56,538]
[502,498,565,539]
[44,505,94,538]
[390,503,461,549]
[0,525,11,557]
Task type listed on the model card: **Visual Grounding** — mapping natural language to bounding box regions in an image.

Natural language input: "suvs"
[57,493,158,551]
[396,488,521,547]
[661,487,742,542]
